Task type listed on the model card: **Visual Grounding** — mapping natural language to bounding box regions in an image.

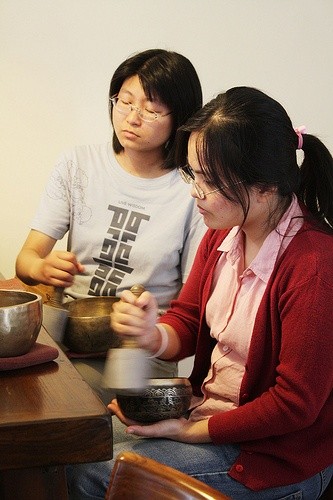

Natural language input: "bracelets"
[144,323,168,361]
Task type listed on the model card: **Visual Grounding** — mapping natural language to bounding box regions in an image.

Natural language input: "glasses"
[178,164,244,199]
[109,94,173,123]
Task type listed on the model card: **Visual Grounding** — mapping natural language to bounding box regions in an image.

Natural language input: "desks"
[0,271,113,500]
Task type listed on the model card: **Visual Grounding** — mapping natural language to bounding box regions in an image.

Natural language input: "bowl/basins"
[0,289,43,357]
[117,375,192,422]
[65,296,129,350]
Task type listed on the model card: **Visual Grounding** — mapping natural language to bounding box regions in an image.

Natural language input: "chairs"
[106,452,233,500]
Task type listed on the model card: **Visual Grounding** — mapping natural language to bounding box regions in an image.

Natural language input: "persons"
[15,50,210,414]
[66,86,333,500]
